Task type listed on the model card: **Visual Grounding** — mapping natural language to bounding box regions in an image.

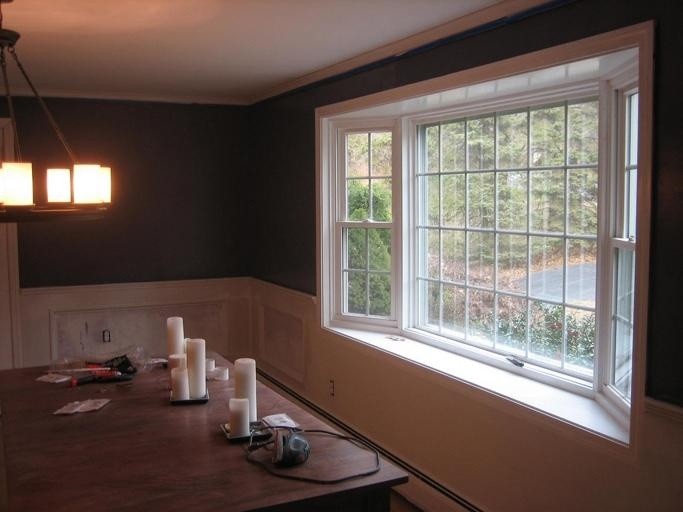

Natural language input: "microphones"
[248,439,275,451]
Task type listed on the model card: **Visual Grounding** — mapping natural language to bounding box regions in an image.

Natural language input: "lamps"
[0,3,116,224]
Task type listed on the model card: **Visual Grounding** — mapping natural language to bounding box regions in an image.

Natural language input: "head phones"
[273,430,311,463]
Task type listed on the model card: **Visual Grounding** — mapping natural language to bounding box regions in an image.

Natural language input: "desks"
[2,350,410,511]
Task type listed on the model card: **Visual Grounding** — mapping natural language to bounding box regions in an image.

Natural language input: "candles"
[166,317,258,438]
